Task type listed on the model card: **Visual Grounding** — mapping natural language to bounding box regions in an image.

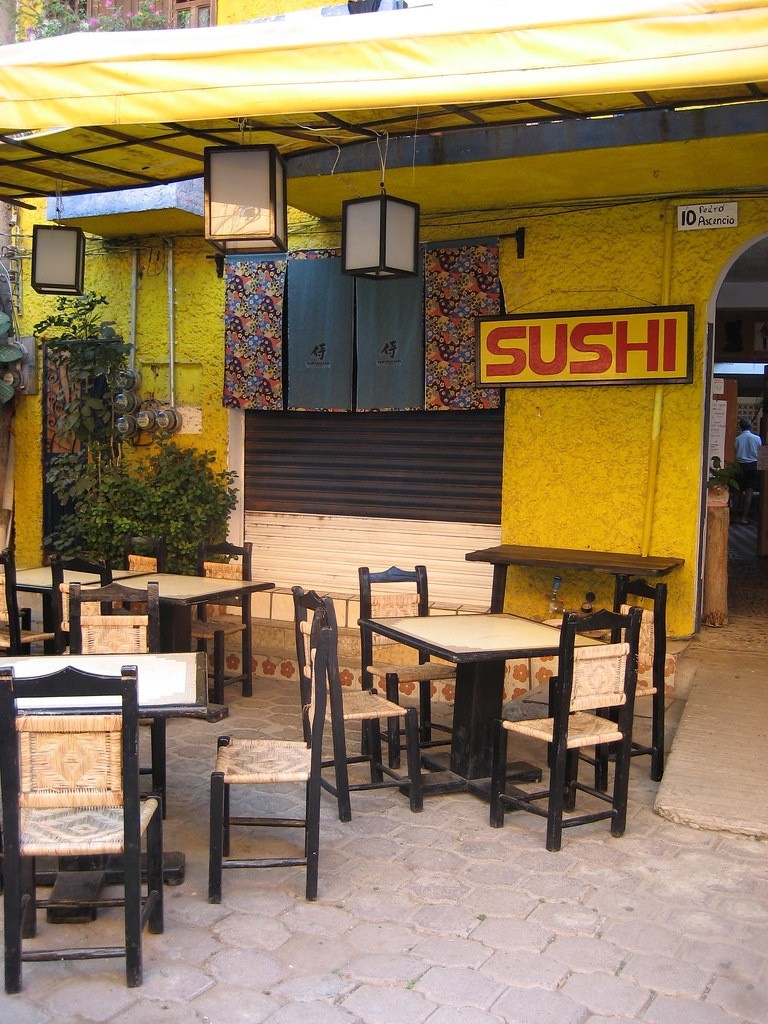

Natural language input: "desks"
[3,652,211,995]
[357,613,607,813]
[14,565,276,722]
[466,544,685,613]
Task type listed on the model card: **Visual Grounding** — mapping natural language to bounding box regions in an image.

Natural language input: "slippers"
[740,520,749,524]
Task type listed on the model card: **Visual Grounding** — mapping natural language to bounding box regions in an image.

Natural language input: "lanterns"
[341,192,419,281]
[31,224,86,296]
[203,145,288,254]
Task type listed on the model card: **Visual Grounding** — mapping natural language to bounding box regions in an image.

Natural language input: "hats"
[739,417,752,426]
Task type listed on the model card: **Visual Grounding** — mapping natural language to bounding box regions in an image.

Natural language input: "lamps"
[32,174,86,295]
[203,117,288,255]
[339,135,418,281]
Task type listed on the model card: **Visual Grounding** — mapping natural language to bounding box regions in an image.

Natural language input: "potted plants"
[708,456,741,496]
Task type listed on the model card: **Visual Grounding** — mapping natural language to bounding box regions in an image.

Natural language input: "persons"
[730,416,762,524]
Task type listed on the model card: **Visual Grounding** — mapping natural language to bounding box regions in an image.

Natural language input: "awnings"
[0,1,768,206]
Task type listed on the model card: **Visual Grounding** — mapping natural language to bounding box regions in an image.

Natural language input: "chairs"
[290,566,670,852]
[1,581,180,915]
[2,536,253,704]
[208,607,325,903]
